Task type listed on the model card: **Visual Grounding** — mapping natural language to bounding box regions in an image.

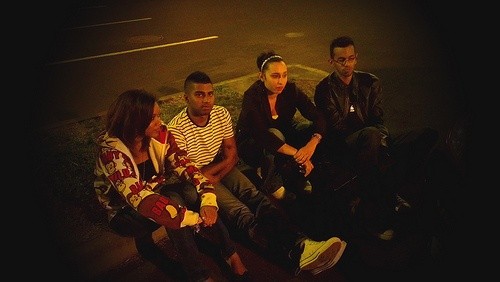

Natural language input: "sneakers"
[311,241,347,275]
[299,237,342,271]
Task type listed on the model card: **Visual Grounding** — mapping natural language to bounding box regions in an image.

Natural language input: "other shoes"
[368,214,395,242]
[227,270,256,282]
[391,190,412,217]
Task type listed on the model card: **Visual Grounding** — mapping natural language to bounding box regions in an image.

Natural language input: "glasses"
[331,55,356,65]
